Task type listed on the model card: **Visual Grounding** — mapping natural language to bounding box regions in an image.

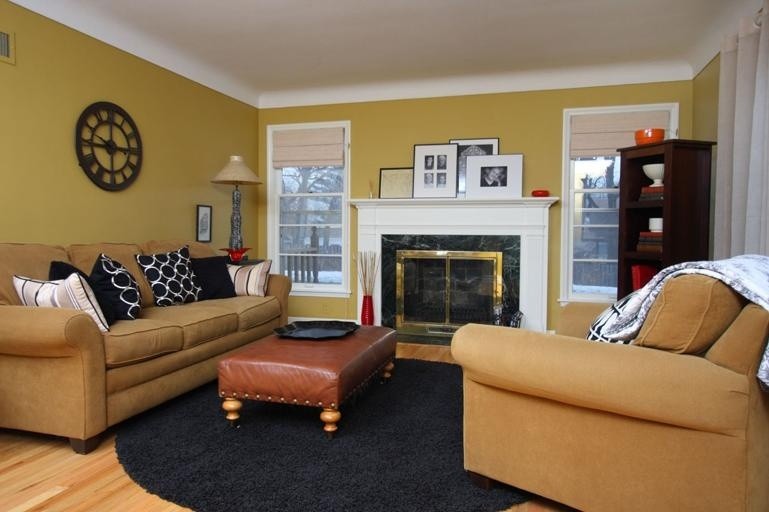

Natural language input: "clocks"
[74,102,143,192]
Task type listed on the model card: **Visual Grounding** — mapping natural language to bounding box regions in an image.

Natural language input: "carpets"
[115,356,538,511]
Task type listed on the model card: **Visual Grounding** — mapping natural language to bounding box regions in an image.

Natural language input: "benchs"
[217,324,397,440]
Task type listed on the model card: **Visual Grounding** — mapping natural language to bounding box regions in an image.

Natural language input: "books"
[632,231,664,292]
[637,187,665,202]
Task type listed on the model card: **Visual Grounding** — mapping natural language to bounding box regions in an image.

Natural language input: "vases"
[360,294,374,325]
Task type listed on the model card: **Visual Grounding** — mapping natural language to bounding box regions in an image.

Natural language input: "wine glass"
[643,163,664,187]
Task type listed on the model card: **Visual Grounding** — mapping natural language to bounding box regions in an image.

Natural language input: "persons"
[424,173,432,188]
[437,156,446,169]
[425,156,433,169]
[437,174,446,187]
[481,168,503,186]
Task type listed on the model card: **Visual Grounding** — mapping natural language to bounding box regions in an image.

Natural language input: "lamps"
[210,155,263,261]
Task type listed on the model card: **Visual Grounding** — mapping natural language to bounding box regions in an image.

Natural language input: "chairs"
[450,274,768,511]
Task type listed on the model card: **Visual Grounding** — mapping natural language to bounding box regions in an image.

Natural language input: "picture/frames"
[379,137,522,199]
[196,204,212,243]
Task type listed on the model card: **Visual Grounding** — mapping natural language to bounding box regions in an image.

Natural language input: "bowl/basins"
[635,128,665,145]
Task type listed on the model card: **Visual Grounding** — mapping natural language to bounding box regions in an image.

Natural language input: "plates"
[648,217,664,233]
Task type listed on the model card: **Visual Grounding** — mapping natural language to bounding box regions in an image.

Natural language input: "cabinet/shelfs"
[616,138,718,304]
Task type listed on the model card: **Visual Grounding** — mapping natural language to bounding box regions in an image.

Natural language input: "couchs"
[0,240,294,455]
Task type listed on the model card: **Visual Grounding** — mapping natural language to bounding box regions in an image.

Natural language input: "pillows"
[10,244,272,332]
